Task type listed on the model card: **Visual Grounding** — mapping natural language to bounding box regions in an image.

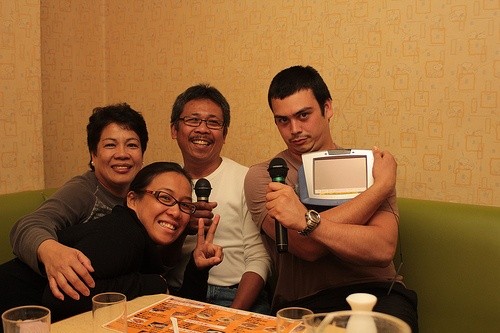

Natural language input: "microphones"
[267,157,289,254]
[193,178,212,239]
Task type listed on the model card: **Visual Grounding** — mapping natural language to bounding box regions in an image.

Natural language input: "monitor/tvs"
[298,148,376,206]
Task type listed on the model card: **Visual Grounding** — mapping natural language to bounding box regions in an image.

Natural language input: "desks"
[52,293,347,333]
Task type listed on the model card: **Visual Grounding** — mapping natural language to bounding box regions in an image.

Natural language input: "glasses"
[178,115,225,130]
[140,189,198,216]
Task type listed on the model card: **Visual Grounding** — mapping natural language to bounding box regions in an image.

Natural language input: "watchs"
[298,210,321,236]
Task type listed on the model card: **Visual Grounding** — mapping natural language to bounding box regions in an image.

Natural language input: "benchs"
[0,189,500,333]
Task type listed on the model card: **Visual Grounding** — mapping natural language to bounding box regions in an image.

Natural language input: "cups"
[92,292,127,333]
[277,307,314,333]
[2,305,51,333]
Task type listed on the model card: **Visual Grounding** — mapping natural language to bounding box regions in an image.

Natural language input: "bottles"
[346,293,380,333]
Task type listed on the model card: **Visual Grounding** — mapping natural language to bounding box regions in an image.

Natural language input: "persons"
[244,65,419,333]
[0,83,279,333]
